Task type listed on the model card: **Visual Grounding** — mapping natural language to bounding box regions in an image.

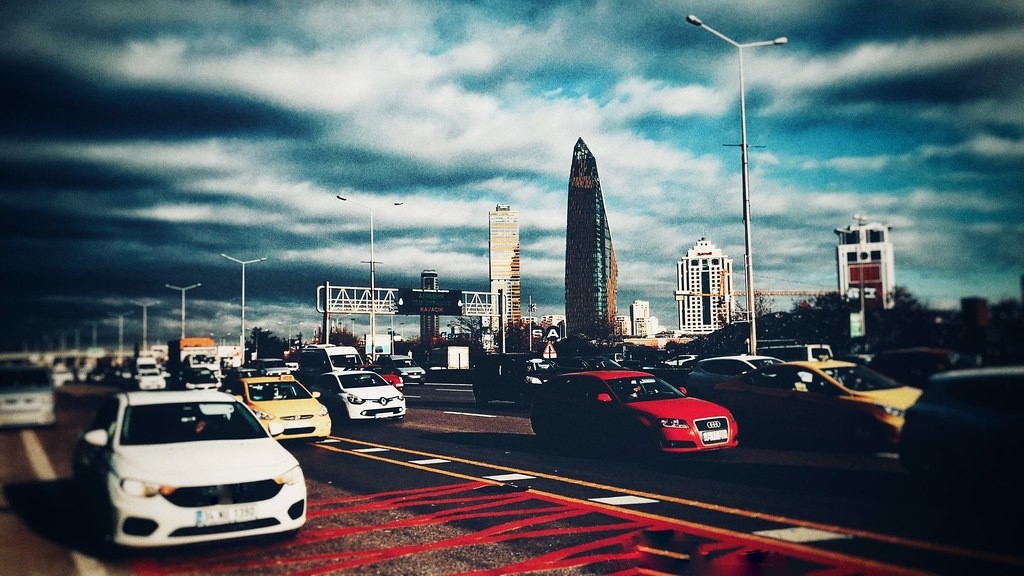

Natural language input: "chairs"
[278,387,291,400]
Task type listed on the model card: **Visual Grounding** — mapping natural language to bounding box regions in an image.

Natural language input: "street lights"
[164,283,202,337]
[219,253,267,366]
[685,13,790,356]
[337,195,404,362]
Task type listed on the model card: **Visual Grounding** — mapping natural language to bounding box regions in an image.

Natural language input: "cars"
[714,353,927,448]
[530,362,744,466]
[0,354,59,430]
[119,343,425,443]
[71,387,311,552]
[468,334,839,420]
